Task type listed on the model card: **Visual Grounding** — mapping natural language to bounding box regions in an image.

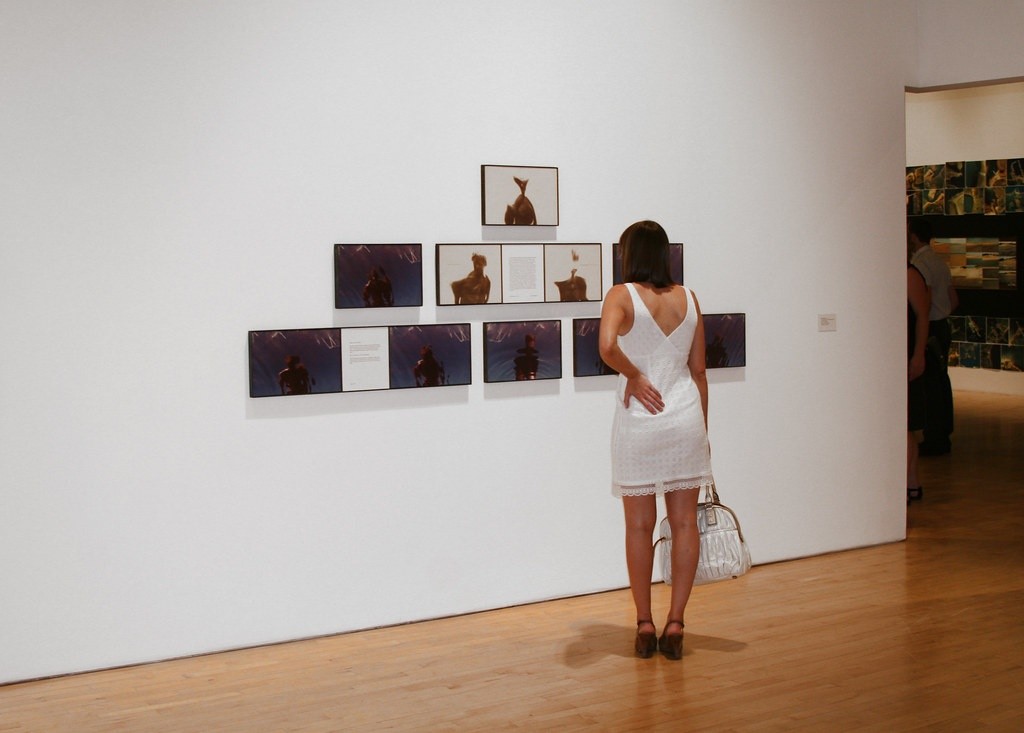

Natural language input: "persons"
[599,220,715,659]
[911,221,959,457]
[907,260,930,506]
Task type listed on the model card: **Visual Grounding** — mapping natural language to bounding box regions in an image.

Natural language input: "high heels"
[635,620,657,658]
[658,620,685,659]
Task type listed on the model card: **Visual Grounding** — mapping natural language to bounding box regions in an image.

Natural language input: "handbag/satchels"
[660,474,751,587]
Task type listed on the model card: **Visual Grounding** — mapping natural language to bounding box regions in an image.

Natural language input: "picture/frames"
[611,243,687,286]
[479,163,561,227]
[483,319,563,383]
[334,243,423,310]
[433,243,604,307]
[246,322,473,403]
[572,317,621,378]
[692,312,746,369]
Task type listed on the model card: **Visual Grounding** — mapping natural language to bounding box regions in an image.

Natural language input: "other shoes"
[920,441,951,455]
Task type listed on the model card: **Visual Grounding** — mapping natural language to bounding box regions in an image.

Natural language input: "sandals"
[907,487,922,504]
